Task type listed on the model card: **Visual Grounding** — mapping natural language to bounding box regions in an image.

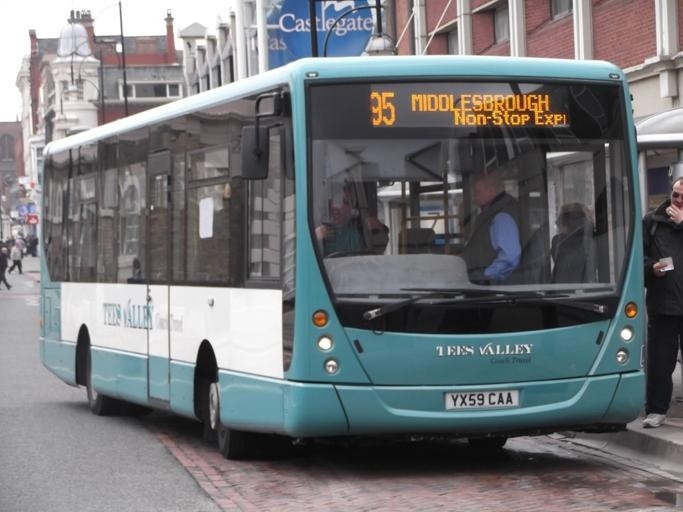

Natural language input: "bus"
[38,53,650,462]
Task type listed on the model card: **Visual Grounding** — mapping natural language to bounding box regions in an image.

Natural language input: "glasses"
[371,226,389,234]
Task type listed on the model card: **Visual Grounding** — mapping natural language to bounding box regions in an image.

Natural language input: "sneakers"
[644,412,666,427]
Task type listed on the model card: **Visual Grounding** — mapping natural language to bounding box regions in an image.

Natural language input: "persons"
[462,173,523,285]
[551,203,596,283]
[0,233,38,289]
[314,191,389,258]
[643,176,683,428]
